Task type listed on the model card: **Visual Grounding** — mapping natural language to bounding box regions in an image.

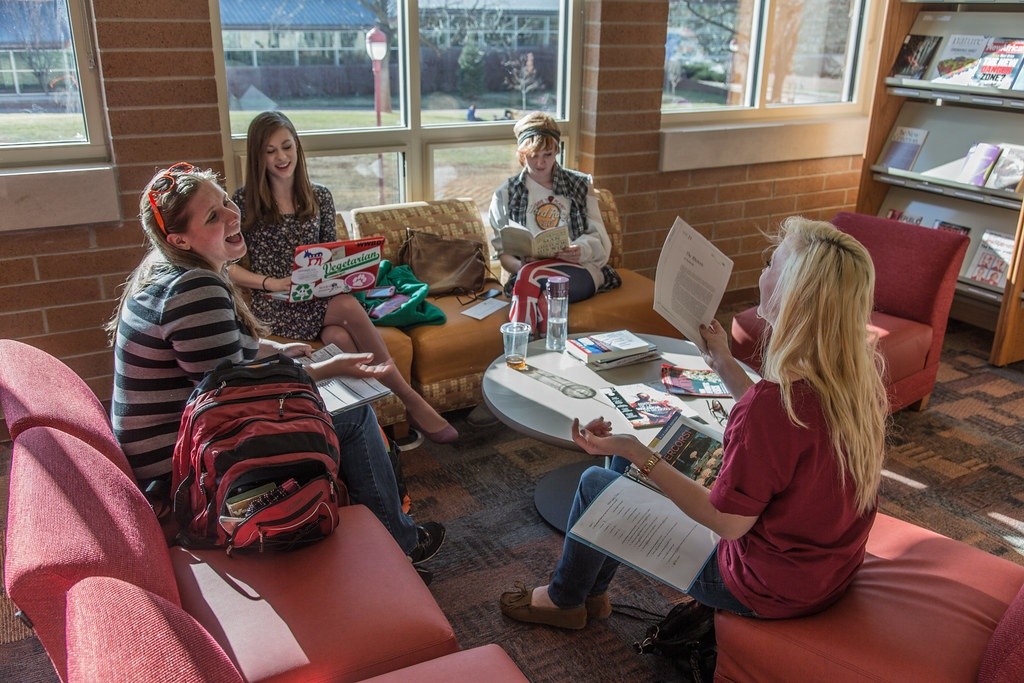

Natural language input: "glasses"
[147,161,196,237]
[705,400,731,424]
[430,286,477,306]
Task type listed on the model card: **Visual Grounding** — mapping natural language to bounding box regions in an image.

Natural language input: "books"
[887,32,942,81]
[657,364,733,398]
[931,34,1024,91]
[288,343,393,415]
[956,141,1024,194]
[600,379,700,429]
[886,208,922,225]
[933,219,971,237]
[500,218,570,262]
[565,329,662,371]
[964,228,1016,289]
[652,215,735,354]
[219,482,276,536]
[880,126,929,172]
[567,412,725,594]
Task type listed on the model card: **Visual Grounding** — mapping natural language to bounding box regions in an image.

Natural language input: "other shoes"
[403,404,459,442]
[500,583,589,631]
[467,396,499,427]
[586,594,614,623]
[406,518,444,566]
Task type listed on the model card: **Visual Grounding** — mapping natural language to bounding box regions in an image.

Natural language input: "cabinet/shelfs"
[855,0,1024,367]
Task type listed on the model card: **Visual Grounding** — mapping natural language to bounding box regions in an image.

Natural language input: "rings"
[572,251,576,256]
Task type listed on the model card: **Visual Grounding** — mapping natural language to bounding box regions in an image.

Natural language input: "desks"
[482,331,762,538]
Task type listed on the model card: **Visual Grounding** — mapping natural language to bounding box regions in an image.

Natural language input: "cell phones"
[477,289,502,299]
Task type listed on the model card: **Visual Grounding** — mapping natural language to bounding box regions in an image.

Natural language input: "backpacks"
[168,354,338,555]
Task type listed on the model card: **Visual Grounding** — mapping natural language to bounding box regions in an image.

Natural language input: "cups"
[546,276,568,352]
[500,322,531,368]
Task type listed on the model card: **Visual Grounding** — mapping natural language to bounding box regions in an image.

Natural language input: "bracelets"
[637,452,662,480]
[262,276,273,292]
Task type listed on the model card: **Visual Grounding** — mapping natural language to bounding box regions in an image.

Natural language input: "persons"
[110,161,447,567]
[231,112,459,444]
[499,215,891,630]
[489,113,612,342]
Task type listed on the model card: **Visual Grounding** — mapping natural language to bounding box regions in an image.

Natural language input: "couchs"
[0,188,684,683]
[713,511,1024,683]
[732,211,971,417]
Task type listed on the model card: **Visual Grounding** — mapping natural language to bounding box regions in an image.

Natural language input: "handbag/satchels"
[632,597,715,682]
[398,226,490,296]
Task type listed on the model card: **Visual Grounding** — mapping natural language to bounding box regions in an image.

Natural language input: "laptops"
[265,236,384,303]
